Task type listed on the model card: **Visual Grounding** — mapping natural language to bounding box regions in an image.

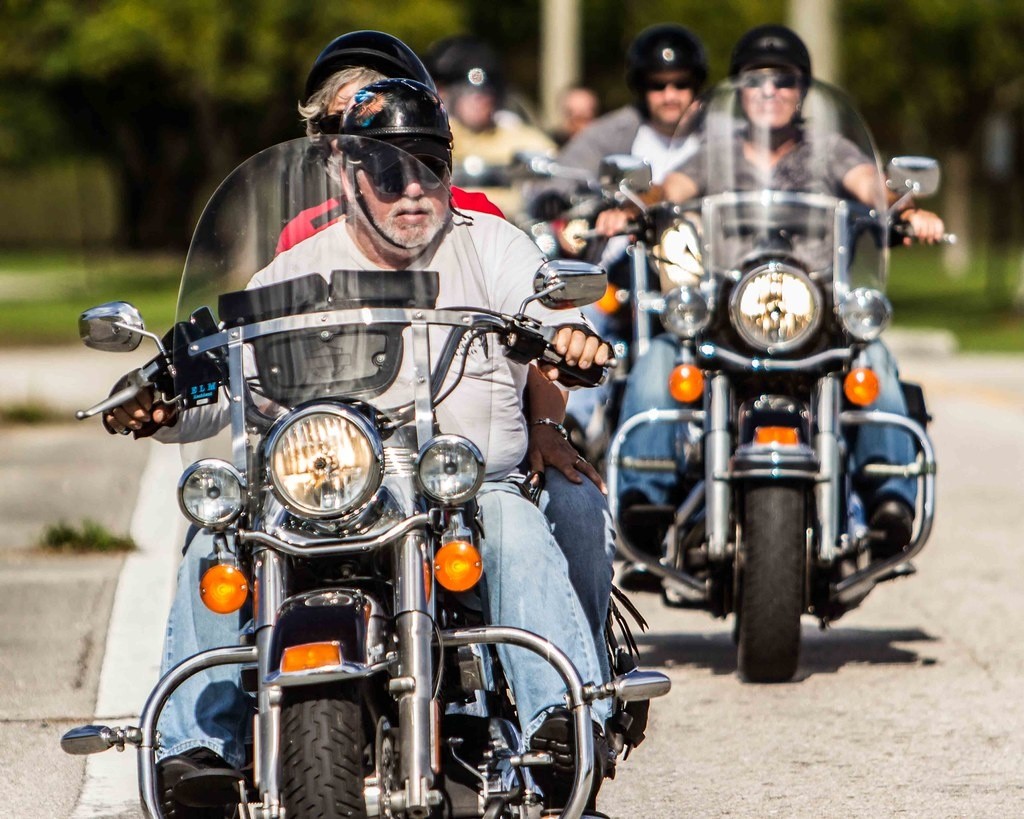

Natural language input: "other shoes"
[619,487,674,559]
[156,747,235,819]
[864,491,915,520]
[530,709,596,813]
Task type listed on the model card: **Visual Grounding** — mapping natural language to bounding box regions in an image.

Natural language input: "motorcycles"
[60,133,675,819]
[443,61,959,684]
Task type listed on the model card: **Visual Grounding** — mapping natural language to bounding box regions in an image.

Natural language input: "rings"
[574,455,586,464]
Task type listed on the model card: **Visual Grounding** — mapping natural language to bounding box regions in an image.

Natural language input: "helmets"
[423,35,498,82]
[336,77,452,175]
[625,22,710,94]
[727,24,812,83]
[305,30,437,99]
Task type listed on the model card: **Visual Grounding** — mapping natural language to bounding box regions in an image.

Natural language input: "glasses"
[372,157,448,195]
[643,77,695,91]
[739,70,797,88]
[309,115,341,134]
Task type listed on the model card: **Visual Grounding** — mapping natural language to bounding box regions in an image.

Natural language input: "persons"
[102,24,945,819]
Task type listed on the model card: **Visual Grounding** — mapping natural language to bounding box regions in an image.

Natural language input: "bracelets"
[527,416,569,440]
